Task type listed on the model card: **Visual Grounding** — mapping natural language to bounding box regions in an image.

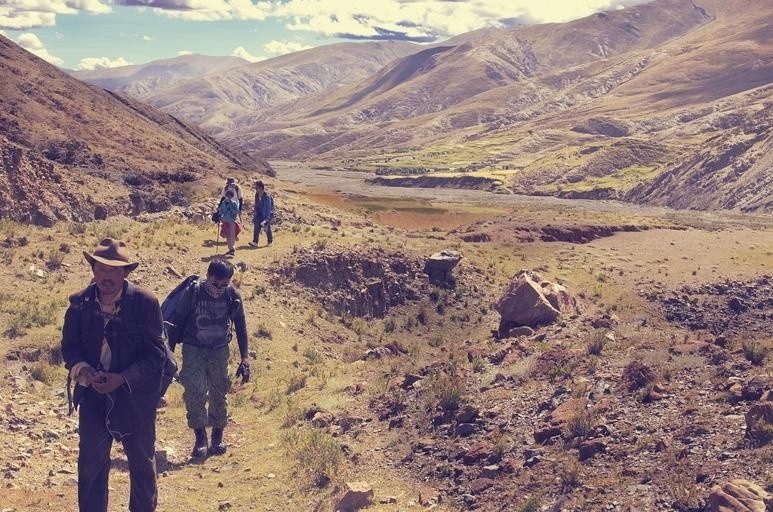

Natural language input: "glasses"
[211,282,229,287]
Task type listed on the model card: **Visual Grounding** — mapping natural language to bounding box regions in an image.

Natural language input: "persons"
[175,258,251,457]
[60,237,167,512]
[217,190,240,257]
[217,176,244,241]
[248,180,274,247]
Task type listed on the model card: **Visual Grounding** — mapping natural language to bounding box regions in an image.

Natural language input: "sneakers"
[249,242,258,247]
[266,242,272,247]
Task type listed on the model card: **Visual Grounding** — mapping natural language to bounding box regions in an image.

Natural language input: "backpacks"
[160,275,232,352]
[66,278,177,416]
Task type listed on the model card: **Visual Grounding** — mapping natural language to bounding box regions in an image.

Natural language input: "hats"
[83,238,139,273]
[226,177,238,185]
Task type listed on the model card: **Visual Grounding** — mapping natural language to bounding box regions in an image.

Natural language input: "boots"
[193,427,209,457]
[212,427,227,454]
